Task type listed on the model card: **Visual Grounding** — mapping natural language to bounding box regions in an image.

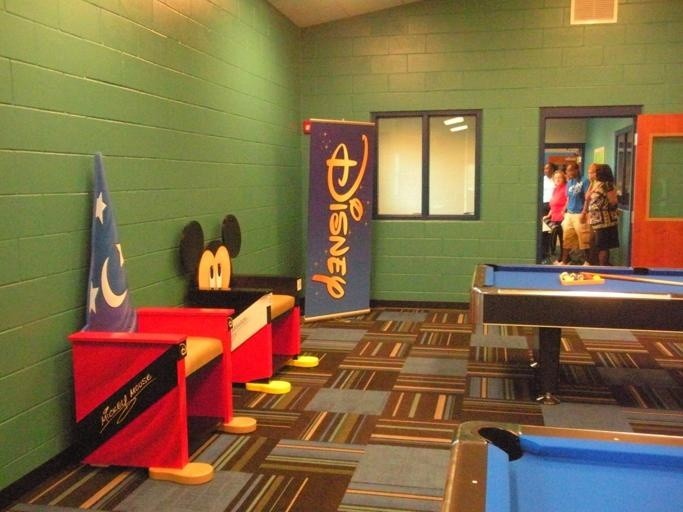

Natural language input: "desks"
[470,262,683,404]
[440,420,683,511]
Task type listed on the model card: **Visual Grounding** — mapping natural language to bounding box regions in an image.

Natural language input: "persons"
[542,162,556,264]
[560,162,594,267]
[578,164,600,224]
[587,164,621,267]
[544,169,567,263]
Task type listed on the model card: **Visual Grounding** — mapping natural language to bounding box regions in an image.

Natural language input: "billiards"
[560,271,602,283]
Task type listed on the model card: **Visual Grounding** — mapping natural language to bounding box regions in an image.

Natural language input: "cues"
[601,274,683,286]
